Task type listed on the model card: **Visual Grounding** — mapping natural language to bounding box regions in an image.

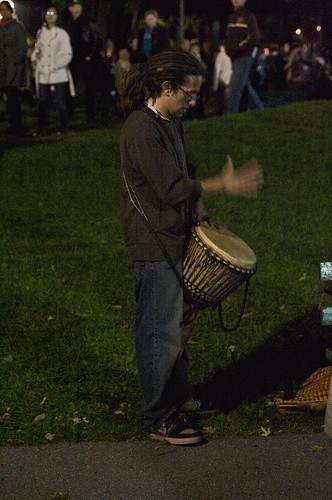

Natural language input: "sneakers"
[178,392,220,414]
[147,412,201,445]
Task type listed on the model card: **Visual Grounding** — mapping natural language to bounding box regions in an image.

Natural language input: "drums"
[182,221,257,345]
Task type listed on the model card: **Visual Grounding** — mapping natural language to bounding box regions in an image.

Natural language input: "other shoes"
[32,130,49,137]
[82,120,96,129]
[56,128,64,135]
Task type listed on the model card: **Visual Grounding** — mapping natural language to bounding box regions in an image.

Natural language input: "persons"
[0,0,332,138]
[118,49,264,446]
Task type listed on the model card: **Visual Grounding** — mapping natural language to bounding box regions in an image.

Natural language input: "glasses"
[179,87,202,103]
